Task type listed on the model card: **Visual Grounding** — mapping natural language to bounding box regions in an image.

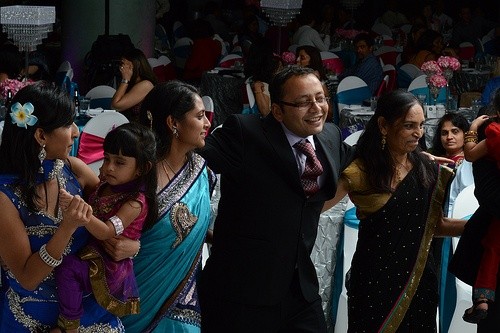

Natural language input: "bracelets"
[37,244,63,269]
[108,215,125,235]
[120,79,129,84]
[129,238,141,260]
[461,131,481,144]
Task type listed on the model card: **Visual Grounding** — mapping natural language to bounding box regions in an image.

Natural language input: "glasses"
[280,96,329,111]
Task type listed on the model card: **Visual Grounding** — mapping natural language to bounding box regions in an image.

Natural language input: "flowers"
[282,52,296,65]
[0,79,26,99]
[10,102,38,130]
[336,28,369,47]
[420,56,461,95]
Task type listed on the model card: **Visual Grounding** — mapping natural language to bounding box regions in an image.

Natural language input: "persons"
[433,113,470,162]
[319,86,467,333]
[198,63,456,333]
[122,80,221,333]
[462,86,500,323]
[0,0,483,126]
[0,79,157,333]
[448,116,500,333]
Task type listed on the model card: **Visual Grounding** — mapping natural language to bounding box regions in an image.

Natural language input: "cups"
[326,68,336,77]
[235,62,244,71]
[445,95,458,114]
[417,93,428,106]
[338,35,384,53]
[471,90,496,120]
[370,96,379,111]
[460,54,495,72]
[79,97,91,114]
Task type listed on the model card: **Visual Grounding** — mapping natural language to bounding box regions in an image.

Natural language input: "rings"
[119,67,124,71]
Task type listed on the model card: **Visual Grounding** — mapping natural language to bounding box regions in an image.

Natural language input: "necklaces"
[160,155,188,181]
[395,167,401,184]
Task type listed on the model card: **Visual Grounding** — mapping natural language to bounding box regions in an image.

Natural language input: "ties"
[292,142,323,199]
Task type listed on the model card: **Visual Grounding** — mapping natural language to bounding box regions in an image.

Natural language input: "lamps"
[0,5,57,79]
[260,0,303,54]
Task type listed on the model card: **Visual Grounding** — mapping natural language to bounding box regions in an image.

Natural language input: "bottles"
[442,19,450,48]
[231,42,243,57]
[64,82,69,99]
[73,83,80,119]
[0,88,13,121]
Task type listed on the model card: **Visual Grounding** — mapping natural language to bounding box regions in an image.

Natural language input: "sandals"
[462,301,489,324]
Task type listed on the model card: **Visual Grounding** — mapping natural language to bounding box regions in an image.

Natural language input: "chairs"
[56,25,500,177]
